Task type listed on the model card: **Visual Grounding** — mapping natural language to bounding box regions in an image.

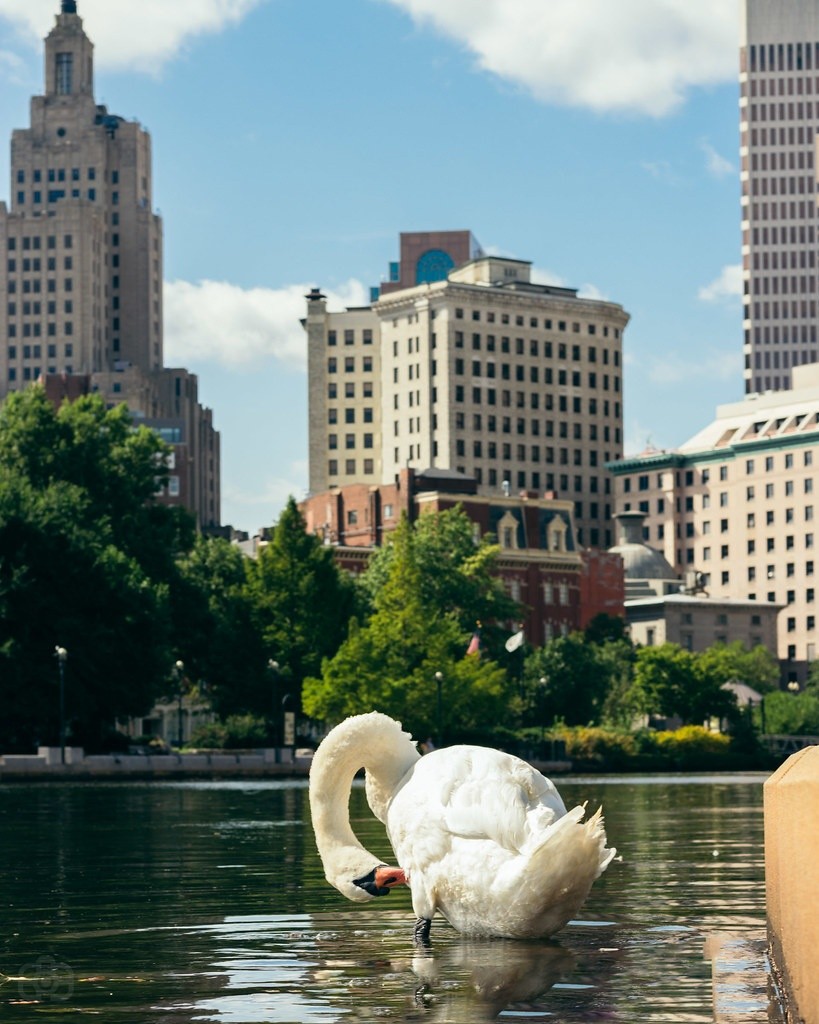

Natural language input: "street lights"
[539,675,546,739]
[434,670,443,733]
[53,645,67,765]
[176,662,184,749]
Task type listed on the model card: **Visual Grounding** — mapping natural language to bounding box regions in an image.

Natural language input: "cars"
[130,733,165,756]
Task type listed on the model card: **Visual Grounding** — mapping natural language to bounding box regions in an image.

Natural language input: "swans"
[307,710,617,947]
